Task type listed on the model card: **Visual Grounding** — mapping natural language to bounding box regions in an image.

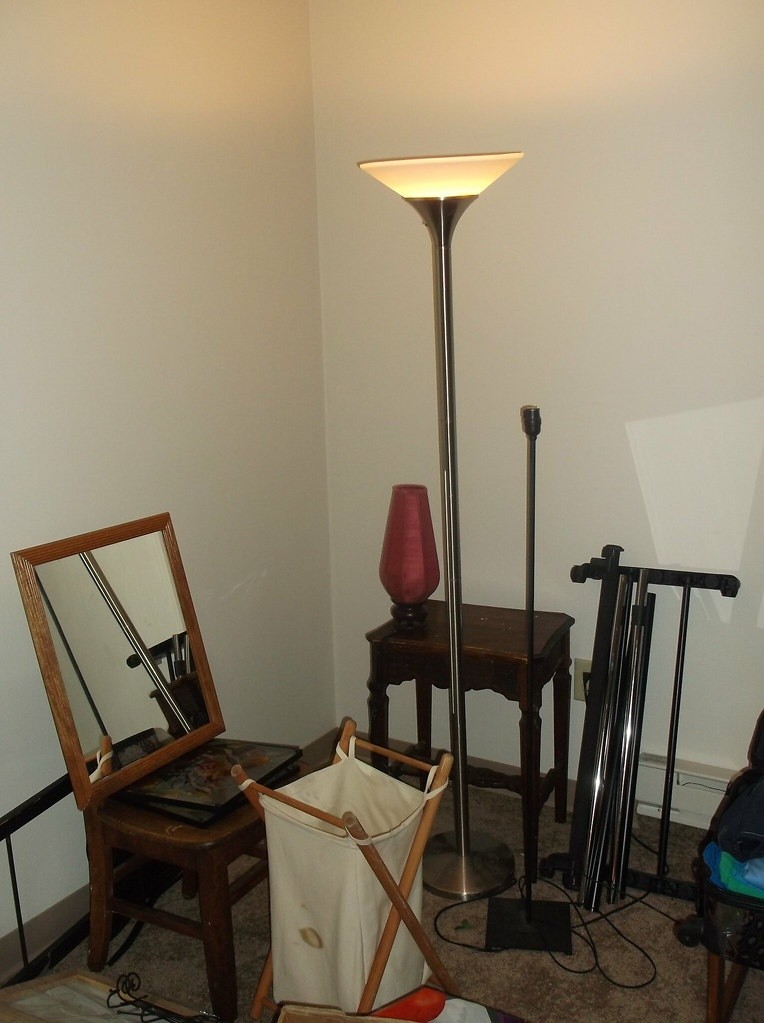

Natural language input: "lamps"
[356,150,554,901]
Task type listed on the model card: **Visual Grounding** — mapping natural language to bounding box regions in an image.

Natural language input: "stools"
[82,762,315,1023]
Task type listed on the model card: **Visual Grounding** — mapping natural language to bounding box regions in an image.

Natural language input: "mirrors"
[11,508,225,812]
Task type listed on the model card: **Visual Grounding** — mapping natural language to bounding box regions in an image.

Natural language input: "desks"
[364,600,576,885]
